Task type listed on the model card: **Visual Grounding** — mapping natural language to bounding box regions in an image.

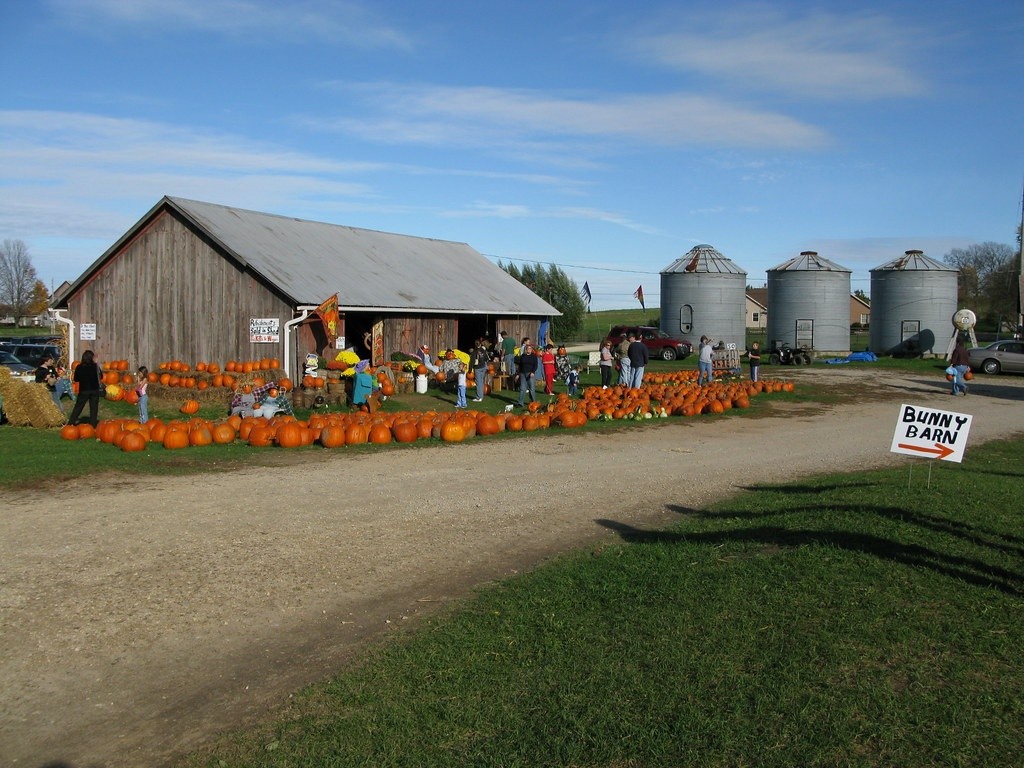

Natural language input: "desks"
[493,375,510,392]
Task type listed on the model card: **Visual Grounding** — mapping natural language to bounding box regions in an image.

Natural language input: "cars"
[967,340,1024,375]
[0,351,37,375]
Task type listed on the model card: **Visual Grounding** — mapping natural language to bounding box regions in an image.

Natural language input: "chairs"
[586,352,602,374]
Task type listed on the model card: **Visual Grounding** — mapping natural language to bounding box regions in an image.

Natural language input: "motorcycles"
[768,342,813,366]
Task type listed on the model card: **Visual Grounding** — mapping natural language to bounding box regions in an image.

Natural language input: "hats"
[700,335,708,343]
[620,333,626,337]
[628,333,635,337]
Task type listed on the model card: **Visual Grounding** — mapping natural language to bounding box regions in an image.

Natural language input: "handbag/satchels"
[97,367,106,397]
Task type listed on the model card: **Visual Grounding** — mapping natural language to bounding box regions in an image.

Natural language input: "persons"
[468,330,582,408]
[948,335,970,396]
[229,382,294,422]
[134,366,149,425]
[599,331,649,389]
[35,355,66,417]
[698,335,725,388]
[55,357,76,401]
[417,344,464,386]
[748,341,760,382]
[64,350,103,432]
[454,362,467,409]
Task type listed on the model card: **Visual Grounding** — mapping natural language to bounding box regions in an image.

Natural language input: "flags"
[315,295,342,345]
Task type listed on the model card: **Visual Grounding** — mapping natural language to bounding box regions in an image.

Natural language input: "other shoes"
[473,399,482,402]
[950,392,954,395]
[963,387,968,396]
[550,393,554,395]
[516,404,521,408]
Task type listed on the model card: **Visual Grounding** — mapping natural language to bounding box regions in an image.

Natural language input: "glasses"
[607,345,609,346]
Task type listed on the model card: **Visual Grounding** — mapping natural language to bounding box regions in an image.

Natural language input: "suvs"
[0,342,60,368]
[607,325,693,361]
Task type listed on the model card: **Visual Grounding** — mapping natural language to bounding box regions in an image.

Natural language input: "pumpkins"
[370,360,445,395]
[634,370,793,421]
[535,350,542,356]
[369,384,667,444]
[557,349,566,355]
[303,376,324,387]
[964,371,973,380]
[465,364,495,395]
[70,360,292,414]
[61,404,372,451]
[946,374,954,381]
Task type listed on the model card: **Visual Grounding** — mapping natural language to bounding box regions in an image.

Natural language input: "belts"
[505,353,513,355]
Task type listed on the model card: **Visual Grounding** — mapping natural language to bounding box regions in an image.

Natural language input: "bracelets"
[950,364,953,366]
